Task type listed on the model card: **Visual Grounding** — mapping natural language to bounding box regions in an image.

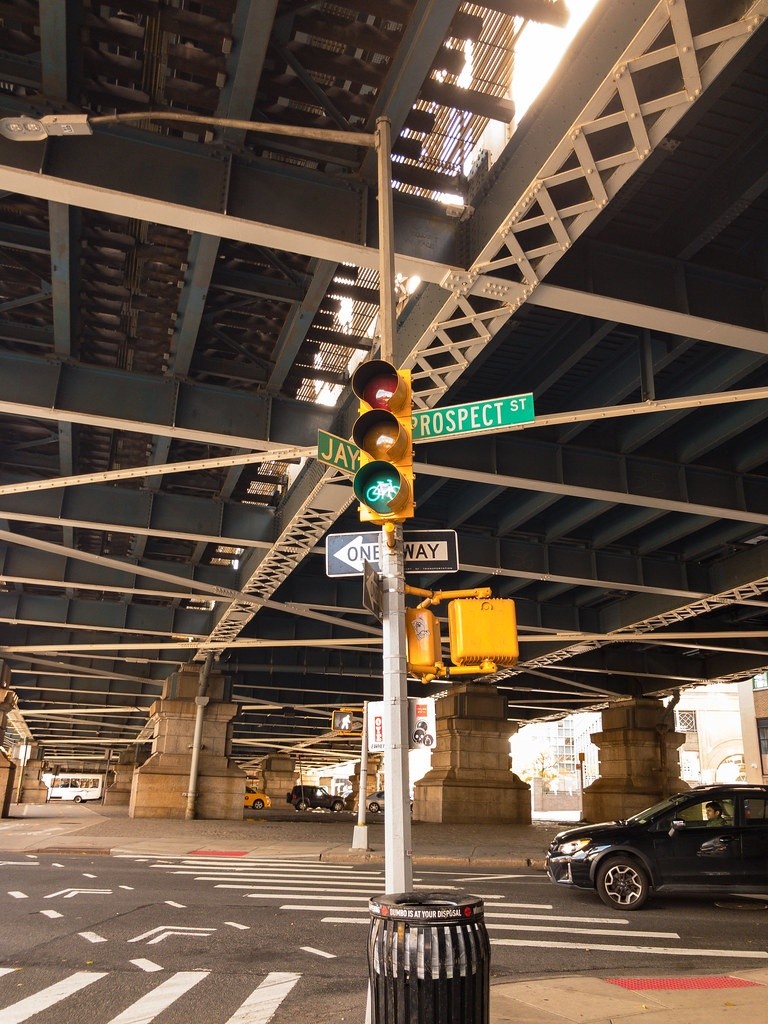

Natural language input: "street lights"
[0,112,412,895]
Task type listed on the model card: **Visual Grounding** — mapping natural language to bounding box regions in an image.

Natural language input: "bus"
[42,772,107,804]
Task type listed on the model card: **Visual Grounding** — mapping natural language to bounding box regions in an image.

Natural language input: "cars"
[245,788,272,809]
[365,791,414,813]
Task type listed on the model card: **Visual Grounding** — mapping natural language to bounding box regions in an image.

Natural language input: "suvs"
[287,785,347,811]
[545,783,768,909]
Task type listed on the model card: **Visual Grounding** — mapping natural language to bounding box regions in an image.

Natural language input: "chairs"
[719,802,734,826]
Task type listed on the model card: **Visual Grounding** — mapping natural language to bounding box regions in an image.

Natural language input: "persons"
[706,802,727,827]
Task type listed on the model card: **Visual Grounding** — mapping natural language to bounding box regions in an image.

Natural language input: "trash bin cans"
[367,890,492,1024]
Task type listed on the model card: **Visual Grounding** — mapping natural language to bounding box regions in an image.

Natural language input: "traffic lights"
[349,360,416,523]
[332,710,354,733]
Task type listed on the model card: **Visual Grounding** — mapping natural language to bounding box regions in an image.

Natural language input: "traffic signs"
[325,530,461,620]
